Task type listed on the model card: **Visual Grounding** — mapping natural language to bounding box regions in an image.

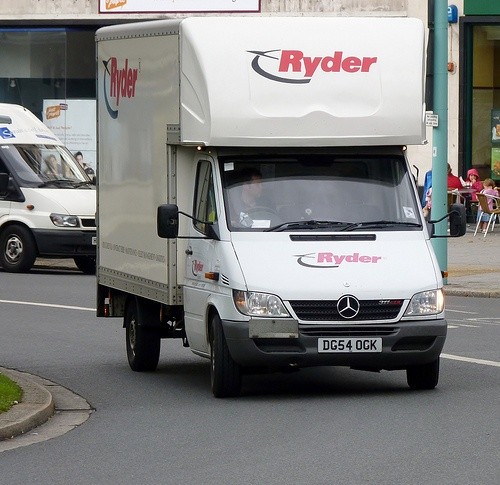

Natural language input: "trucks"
[95,16,448,398]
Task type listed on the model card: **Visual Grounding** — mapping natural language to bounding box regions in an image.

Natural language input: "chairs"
[473,193,500,237]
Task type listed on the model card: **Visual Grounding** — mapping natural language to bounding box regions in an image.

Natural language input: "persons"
[207,169,263,223]
[423,163,499,234]
[69,151,95,175]
[42,154,62,175]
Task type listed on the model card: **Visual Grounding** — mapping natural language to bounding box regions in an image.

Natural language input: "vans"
[0,103,96,274]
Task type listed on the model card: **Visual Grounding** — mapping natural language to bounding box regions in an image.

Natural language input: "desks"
[453,188,476,222]
